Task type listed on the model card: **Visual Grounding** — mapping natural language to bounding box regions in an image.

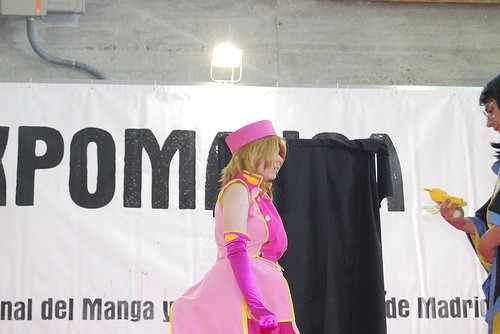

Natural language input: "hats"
[225,121,276,154]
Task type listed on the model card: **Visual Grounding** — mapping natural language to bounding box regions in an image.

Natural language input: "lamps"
[209,42,243,83]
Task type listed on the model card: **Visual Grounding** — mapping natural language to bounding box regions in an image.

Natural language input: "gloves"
[222,231,279,334]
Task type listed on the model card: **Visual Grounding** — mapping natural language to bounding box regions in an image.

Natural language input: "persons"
[437,71,500,334]
[170,120,302,334]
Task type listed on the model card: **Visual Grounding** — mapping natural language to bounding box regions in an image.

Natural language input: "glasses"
[483,100,496,118]
